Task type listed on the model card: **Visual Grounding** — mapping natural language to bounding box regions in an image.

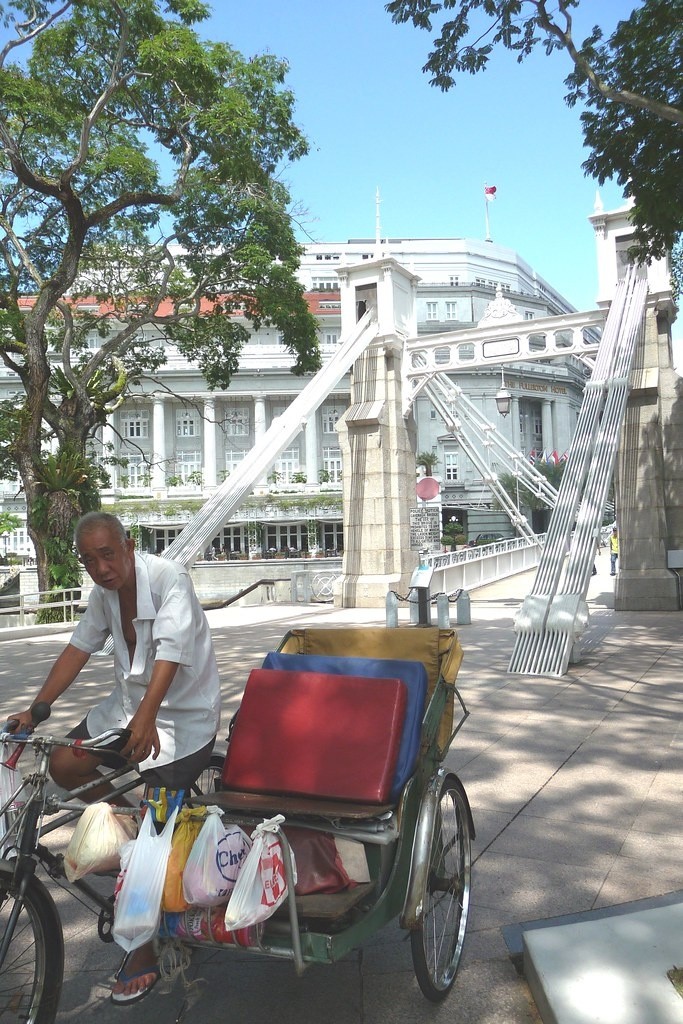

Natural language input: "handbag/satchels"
[65,796,299,956]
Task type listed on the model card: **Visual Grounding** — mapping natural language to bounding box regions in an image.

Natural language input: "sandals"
[111,965,162,1006]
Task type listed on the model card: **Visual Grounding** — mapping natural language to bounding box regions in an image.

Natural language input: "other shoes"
[592,571,596,575]
[610,572,615,575]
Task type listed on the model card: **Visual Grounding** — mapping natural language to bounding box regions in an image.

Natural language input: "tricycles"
[0,626,477,1024]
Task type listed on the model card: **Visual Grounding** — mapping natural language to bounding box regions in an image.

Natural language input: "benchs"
[184,625,464,921]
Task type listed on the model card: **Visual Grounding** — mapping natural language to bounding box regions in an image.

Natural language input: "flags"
[485,186,496,202]
[529,445,568,466]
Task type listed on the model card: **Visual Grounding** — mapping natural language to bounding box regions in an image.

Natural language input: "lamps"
[494,364,512,418]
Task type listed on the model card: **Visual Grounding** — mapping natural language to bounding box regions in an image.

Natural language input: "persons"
[6,513,220,1006]
[266,546,276,559]
[212,545,218,561]
[610,526,618,575]
[284,544,296,559]
[592,533,601,576]
